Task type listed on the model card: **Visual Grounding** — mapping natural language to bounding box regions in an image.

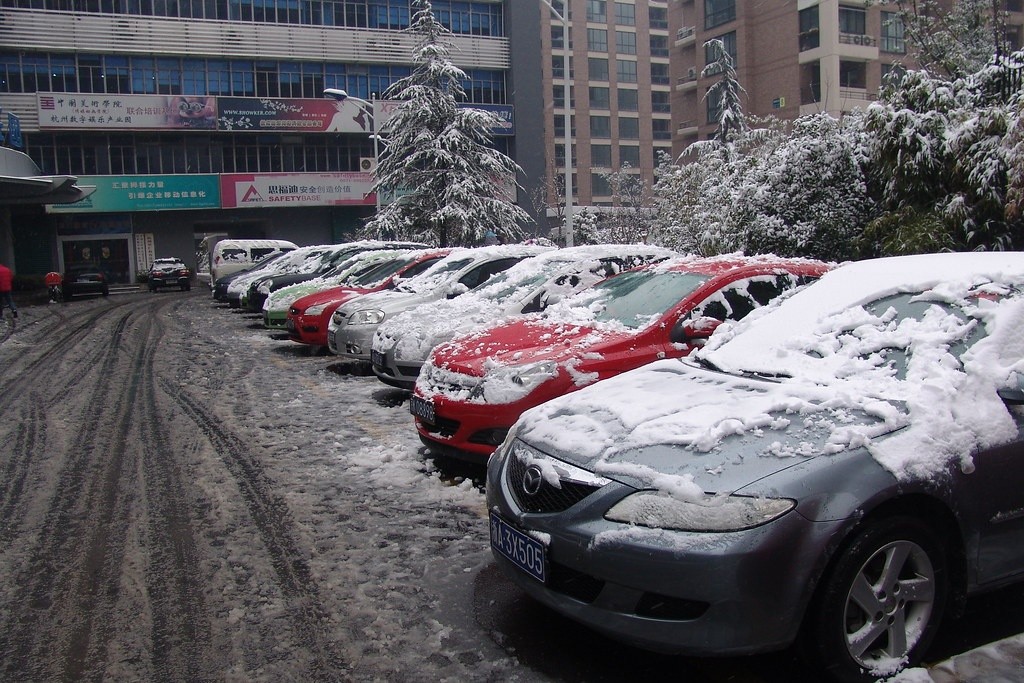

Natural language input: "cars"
[412,254,854,470]
[481,248,1024,683]
[148,257,192,293]
[286,247,469,348]
[326,244,558,362]
[212,243,434,314]
[260,246,416,334]
[369,244,685,393]
[62,264,109,302]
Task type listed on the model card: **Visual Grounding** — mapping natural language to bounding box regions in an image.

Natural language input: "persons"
[0,263,17,318]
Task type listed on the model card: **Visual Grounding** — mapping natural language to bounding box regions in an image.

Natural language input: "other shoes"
[0,313,3,316]
[13,310,18,318]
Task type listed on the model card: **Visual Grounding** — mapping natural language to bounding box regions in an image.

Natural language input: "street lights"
[322,88,383,242]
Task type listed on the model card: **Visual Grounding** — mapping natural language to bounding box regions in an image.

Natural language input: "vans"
[210,239,301,294]
